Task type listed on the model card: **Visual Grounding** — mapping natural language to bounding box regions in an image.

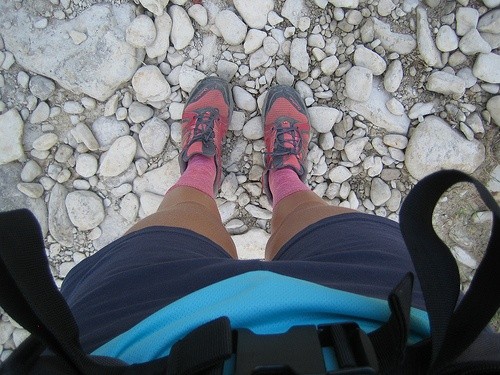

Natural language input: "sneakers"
[262,85,311,206]
[178,76,234,196]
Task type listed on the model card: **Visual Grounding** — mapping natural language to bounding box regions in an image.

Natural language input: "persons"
[0,72,500,375]
